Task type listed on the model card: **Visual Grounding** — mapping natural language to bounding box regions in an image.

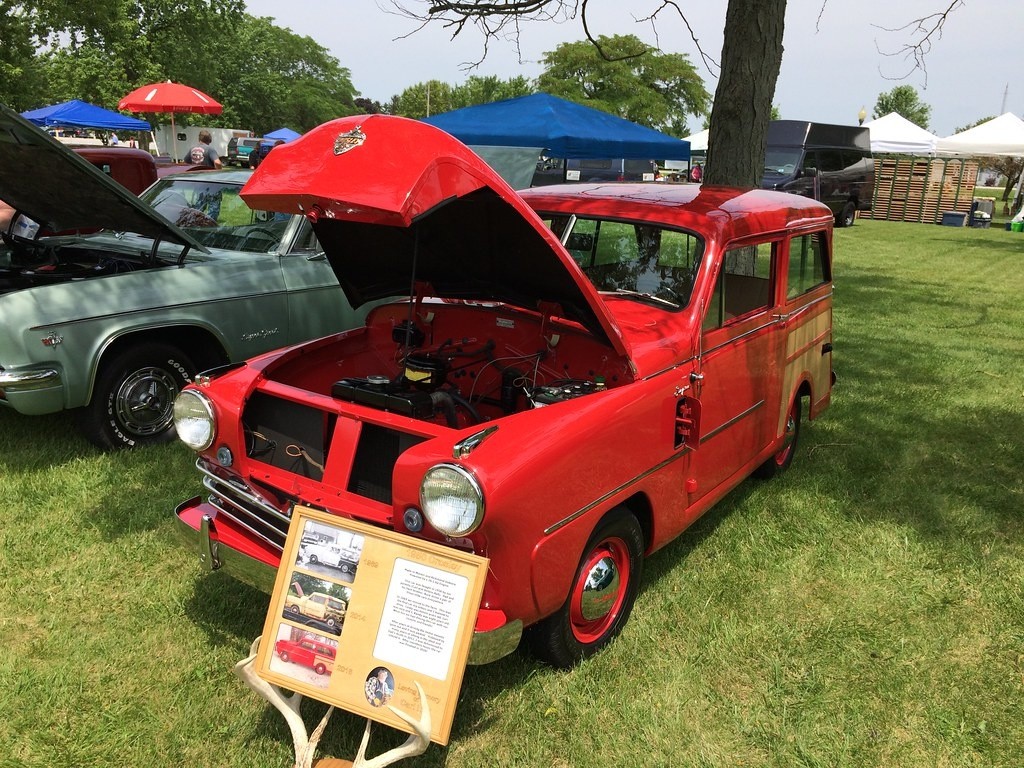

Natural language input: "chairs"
[974,201,993,230]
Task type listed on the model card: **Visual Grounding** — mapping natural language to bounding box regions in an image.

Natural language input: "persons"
[249,147,260,170]
[692,162,702,183]
[0,200,22,231]
[110,132,118,146]
[130,136,137,149]
[183,130,222,170]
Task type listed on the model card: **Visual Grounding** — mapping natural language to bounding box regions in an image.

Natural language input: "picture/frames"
[249,504,491,746]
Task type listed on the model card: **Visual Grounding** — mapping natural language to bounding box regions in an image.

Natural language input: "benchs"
[602,263,769,332]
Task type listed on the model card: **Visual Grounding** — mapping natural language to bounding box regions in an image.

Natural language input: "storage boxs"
[942,210,966,227]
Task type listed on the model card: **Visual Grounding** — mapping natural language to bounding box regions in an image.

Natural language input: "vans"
[762,120,876,227]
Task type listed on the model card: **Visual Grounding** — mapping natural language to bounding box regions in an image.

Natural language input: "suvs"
[48,130,103,146]
[531,156,657,188]
[228,136,268,168]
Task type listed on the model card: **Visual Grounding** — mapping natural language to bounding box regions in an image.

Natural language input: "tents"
[19,98,161,158]
[419,92,709,181]
[859,111,1024,206]
[263,128,301,143]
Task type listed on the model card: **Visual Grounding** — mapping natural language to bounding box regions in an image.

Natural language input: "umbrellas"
[118,79,223,164]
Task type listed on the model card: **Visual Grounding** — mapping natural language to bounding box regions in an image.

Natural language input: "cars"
[0,147,206,272]
[0,103,552,457]
[170,109,836,671]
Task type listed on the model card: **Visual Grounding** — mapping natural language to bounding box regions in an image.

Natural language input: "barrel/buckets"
[1011,222,1022,232]
[1006,222,1011,230]
